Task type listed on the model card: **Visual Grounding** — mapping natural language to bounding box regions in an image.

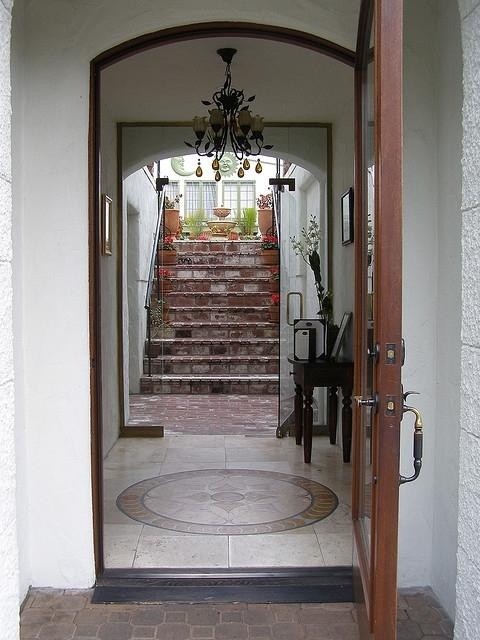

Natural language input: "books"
[293,326,317,364]
[293,318,328,361]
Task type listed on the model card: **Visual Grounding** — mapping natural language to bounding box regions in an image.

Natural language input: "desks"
[287,353,354,463]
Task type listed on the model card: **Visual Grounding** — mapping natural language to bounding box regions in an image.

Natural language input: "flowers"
[289,214,335,323]
[159,236,178,250]
[158,268,168,280]
[270,265,279,281]
[272,293,279,305]
[261,235,278,251]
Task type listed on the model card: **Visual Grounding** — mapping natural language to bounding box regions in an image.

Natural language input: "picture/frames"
[330,312,353,362]
[341,187,354,246]
[102,194,112,256]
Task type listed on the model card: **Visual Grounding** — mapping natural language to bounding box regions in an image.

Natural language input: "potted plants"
[165,194,183,237]
[256,186,272,237]
[150,297,171,335]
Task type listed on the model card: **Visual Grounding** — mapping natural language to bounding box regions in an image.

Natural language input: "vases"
[270,279,279,292]
[157,279,171,290]
[261,249,279,265]
[158,250,177,265]
[268,306,279,322]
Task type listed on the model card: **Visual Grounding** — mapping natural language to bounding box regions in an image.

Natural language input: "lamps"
[185,47,274,181]
